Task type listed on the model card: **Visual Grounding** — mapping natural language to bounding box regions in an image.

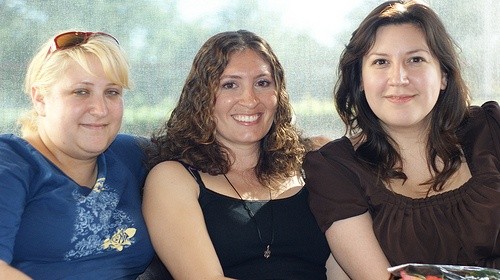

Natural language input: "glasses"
[30,32,119,95]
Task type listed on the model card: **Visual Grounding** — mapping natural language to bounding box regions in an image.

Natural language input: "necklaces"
[221,170,276,258]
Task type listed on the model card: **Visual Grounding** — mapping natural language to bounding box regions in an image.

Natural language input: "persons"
[303,0,500,280]
[141,32,352,280]
[0,31,337,280]
